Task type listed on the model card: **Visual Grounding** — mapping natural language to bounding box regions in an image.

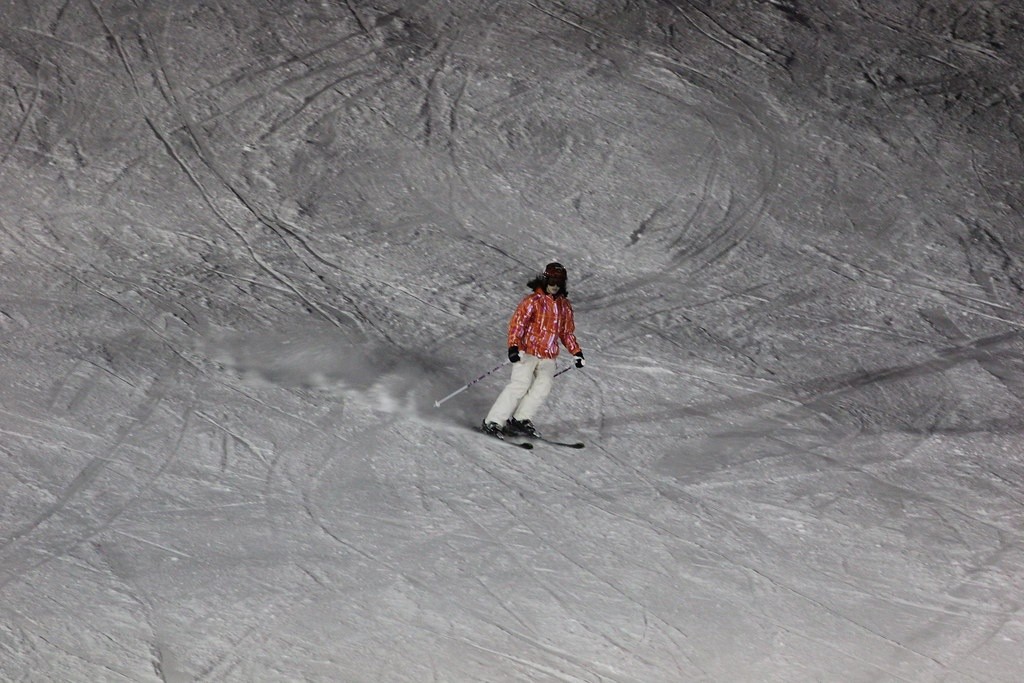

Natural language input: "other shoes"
[513,416,543,437]
[485,418,504,439]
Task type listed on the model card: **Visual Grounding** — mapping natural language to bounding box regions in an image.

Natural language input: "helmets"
[543,263,567,280]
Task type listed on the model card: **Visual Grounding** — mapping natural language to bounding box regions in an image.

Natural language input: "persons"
[482,263,585,439]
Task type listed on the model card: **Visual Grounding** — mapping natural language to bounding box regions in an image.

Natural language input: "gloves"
[508,346,520,363]
[574,351,585,368]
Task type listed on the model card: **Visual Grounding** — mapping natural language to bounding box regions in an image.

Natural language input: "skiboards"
[472,419,584,450]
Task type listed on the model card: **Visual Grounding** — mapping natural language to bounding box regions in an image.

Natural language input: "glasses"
[549,276,565,287]
